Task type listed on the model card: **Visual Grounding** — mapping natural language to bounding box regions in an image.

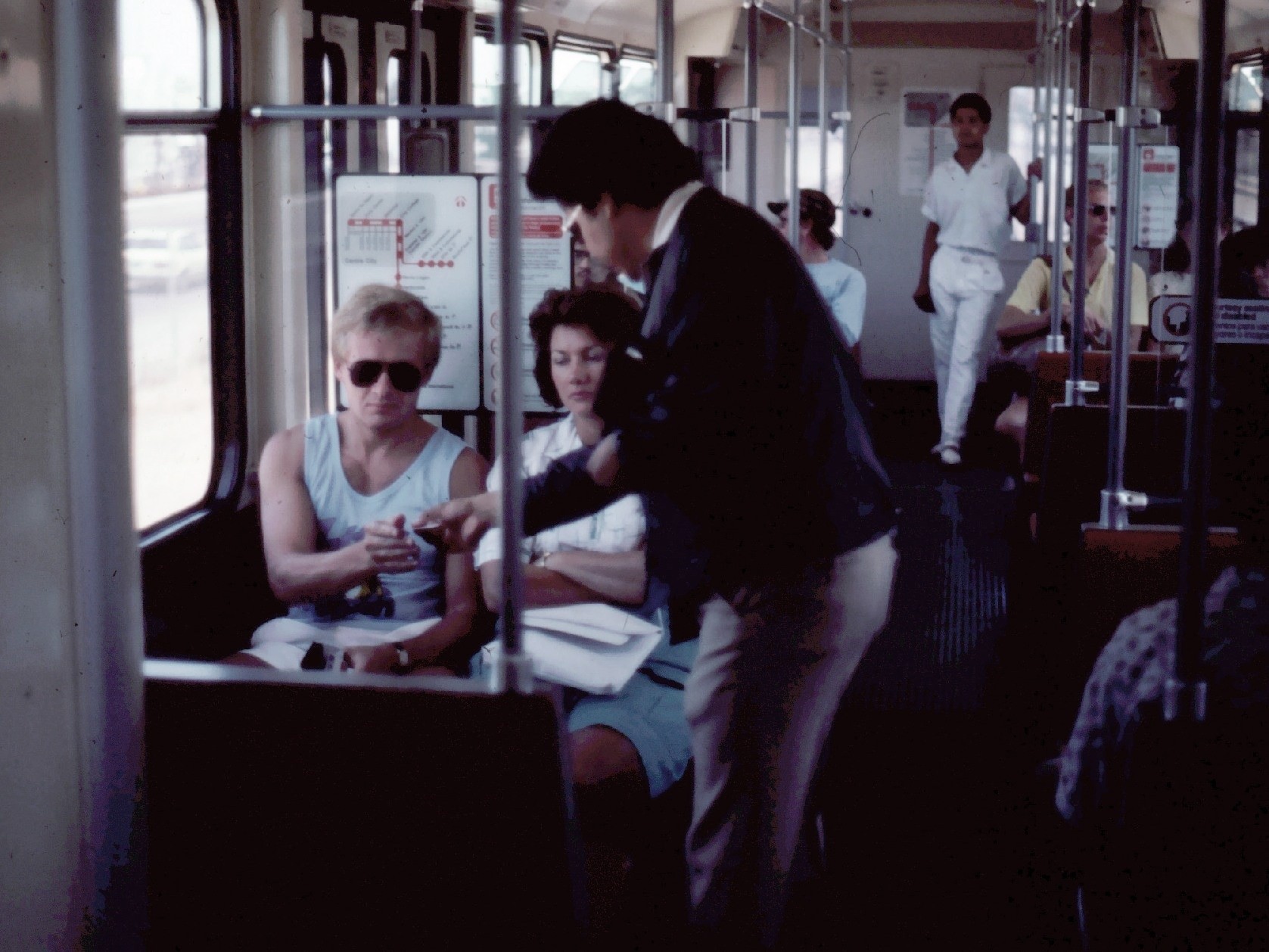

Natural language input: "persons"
[412,96,896,920]
[1056,185,1269,952]
[764,184,866,349]
[215,283,490,682]
[913,91,1041,467]
[988,174,1150,533]
[471,287,707,802]
[565,218,646,312]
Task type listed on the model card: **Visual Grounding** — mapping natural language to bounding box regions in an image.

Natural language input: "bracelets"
[538,552,553,570]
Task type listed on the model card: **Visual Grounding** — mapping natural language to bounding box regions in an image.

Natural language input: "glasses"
[563,202,584,245]
[1088,205,1117,216]
[337,359,422,392]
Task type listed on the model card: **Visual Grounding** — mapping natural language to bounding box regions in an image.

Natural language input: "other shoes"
[941,447,961,465]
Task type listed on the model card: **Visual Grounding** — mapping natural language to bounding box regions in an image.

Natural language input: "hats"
[768,188,836,229]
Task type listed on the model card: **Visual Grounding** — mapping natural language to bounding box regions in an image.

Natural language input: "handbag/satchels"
[497,602,664,696]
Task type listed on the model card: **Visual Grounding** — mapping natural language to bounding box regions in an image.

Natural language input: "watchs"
[390,639,408,675]
